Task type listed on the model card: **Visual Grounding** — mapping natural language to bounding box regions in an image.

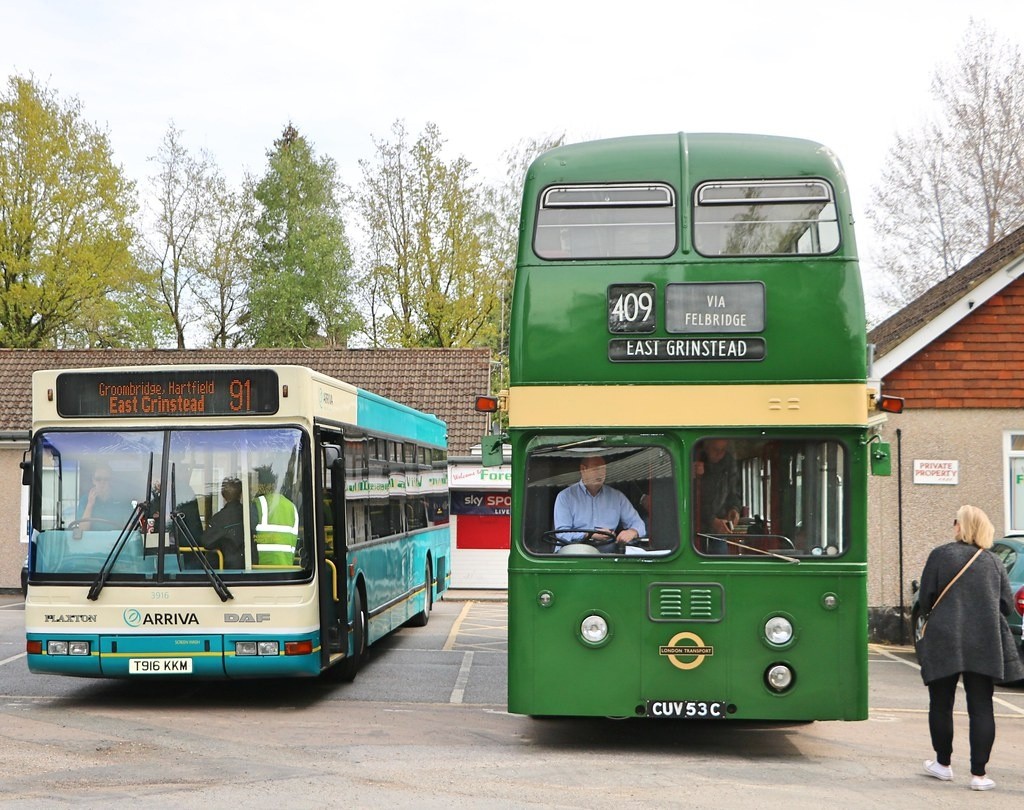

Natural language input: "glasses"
[94,477,109,481]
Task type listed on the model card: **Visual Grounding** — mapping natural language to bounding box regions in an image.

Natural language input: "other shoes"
[971,776,996,791]
[922,760,953,780]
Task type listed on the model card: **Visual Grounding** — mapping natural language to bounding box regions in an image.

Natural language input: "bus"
[476,132,903,721]
[19,364,452,682]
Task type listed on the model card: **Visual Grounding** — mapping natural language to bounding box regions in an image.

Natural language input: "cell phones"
[726,521,734,532]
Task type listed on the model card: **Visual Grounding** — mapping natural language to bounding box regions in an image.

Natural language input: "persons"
[554,455,647,554]
[250,466,299,570]
[78,464,134,531]
[696,440,741,554]
[201,477,258,570]
[913,506,1024,791]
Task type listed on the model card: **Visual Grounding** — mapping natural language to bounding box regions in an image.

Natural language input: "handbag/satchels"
[915,614,930,643]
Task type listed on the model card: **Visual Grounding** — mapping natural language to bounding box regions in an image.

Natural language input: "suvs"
[911,534,1024,688]
